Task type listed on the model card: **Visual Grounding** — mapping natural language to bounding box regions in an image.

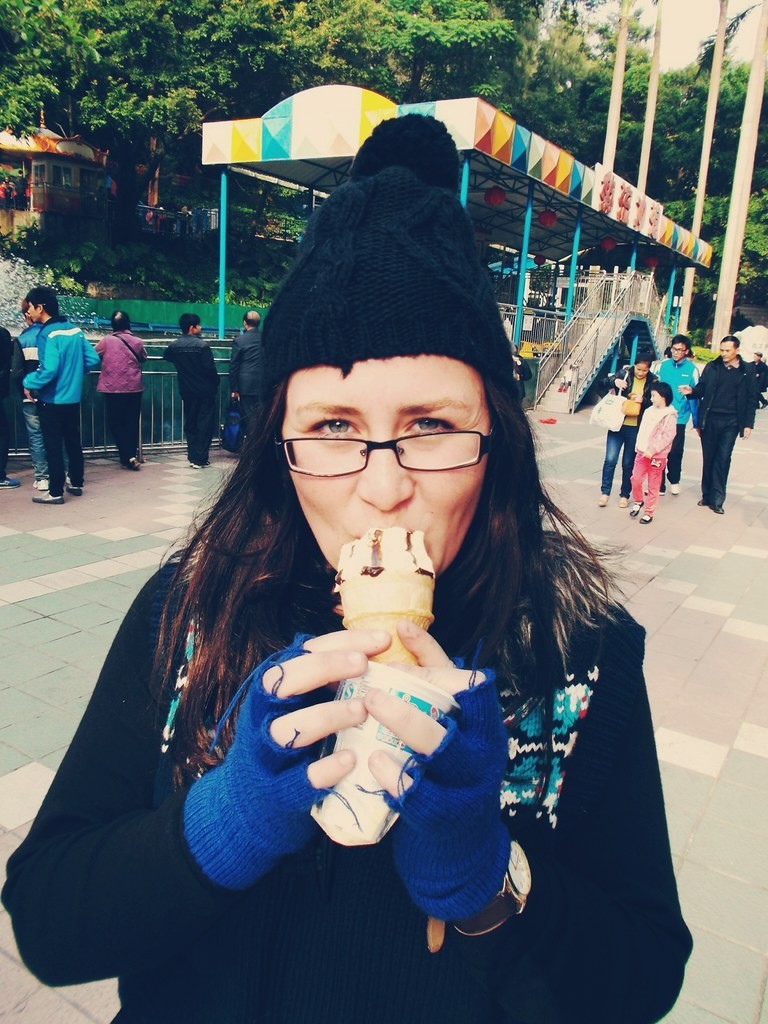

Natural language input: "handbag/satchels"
[589,370,630,431]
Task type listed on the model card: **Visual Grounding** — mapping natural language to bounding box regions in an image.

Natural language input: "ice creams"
[332,527,435,666]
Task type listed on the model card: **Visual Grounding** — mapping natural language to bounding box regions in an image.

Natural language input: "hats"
[259,115,514,385]
[754,351,762,357]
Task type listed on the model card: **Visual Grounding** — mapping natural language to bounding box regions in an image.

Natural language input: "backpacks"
[221,397,243,452]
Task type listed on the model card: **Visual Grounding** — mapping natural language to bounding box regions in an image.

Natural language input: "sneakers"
[0,478,20,489]
[33,479,49,490]
[65,477,70,483]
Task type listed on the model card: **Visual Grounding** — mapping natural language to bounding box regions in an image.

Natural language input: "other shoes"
[639,514,653,524]
[670,484,679,494]
[697,499,710,506]
[619,497,628,508]
[644,489,665,496]
[66,484,82,496]
[122,457,139,470]
[761,400,768,409]
[599,495,609,507]
[710,504,724,514]
[190,461,210,469]
[630,501,644,516]
[32,490,65,504]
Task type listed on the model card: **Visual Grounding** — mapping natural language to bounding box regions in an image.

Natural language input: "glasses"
[671,347,686,352]
[274,417,499,476]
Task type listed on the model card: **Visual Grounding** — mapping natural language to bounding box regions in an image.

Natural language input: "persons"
[750,352,768,409]
[679,336,758,513]
[630,382,678,524]
[147,204,192,229]
[229,311,263,435]
[95,312,147,469]
[23,287,100,504]
[0,326,21,489]
[598,346,660,508]
[0,170,30,211]
[164,313,219,469]
[13,299,70,490]
[0,113,694,1024]
[511,345,532,403]
[653,335,702,495]
